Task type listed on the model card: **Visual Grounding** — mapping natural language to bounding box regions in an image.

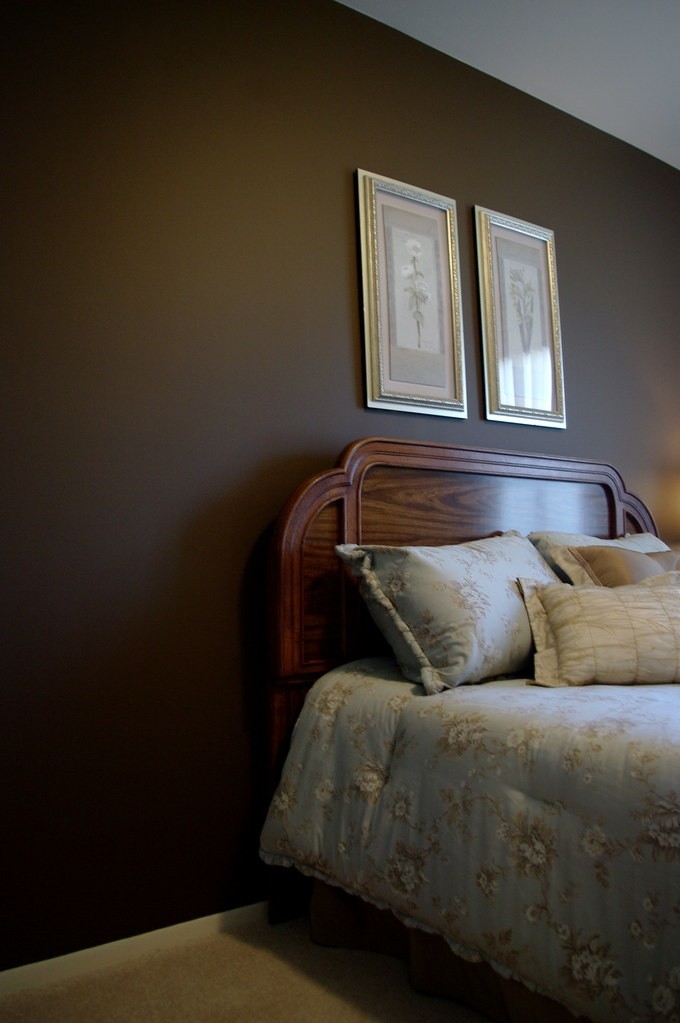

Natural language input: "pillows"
[546,543,680,589]
[516,571,680,687]
[334,528,564,697]
[527,531,671,585]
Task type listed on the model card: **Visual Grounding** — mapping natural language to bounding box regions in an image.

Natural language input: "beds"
[255,438,680,1023]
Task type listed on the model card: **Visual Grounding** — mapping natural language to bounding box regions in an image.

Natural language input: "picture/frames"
[356,169,467,420]
[474,204,566,429]
